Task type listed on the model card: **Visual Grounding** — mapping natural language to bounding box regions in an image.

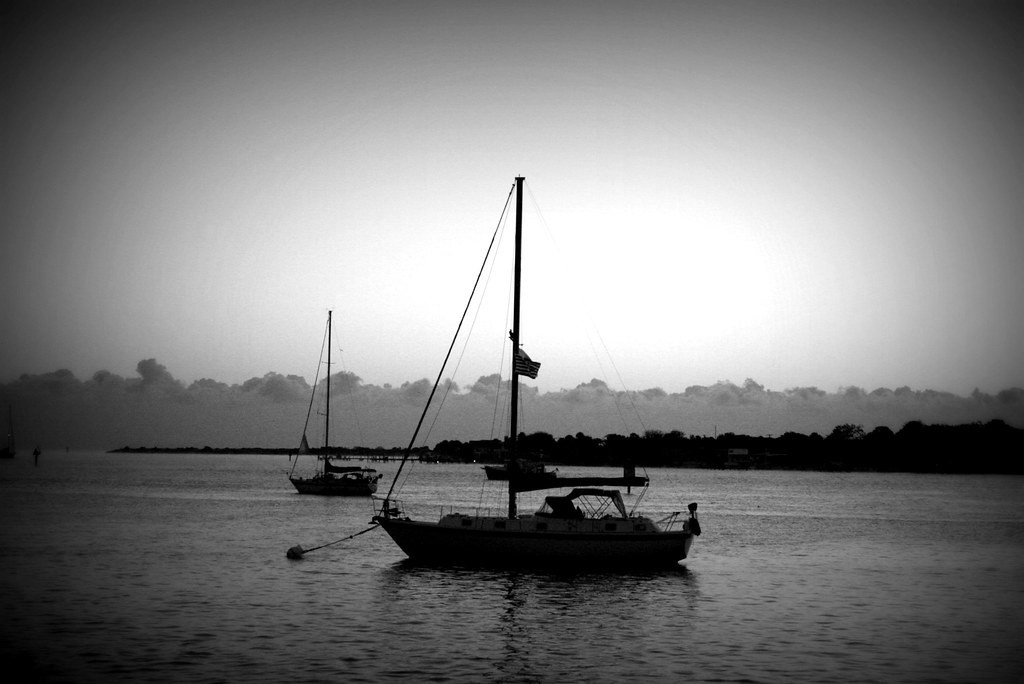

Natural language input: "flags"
[515,347,542,379]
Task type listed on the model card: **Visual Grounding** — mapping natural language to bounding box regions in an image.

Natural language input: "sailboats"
[369,172,702,577]
[288,311,383,497]
[479,261,549,482]
[0,403,17,460]
